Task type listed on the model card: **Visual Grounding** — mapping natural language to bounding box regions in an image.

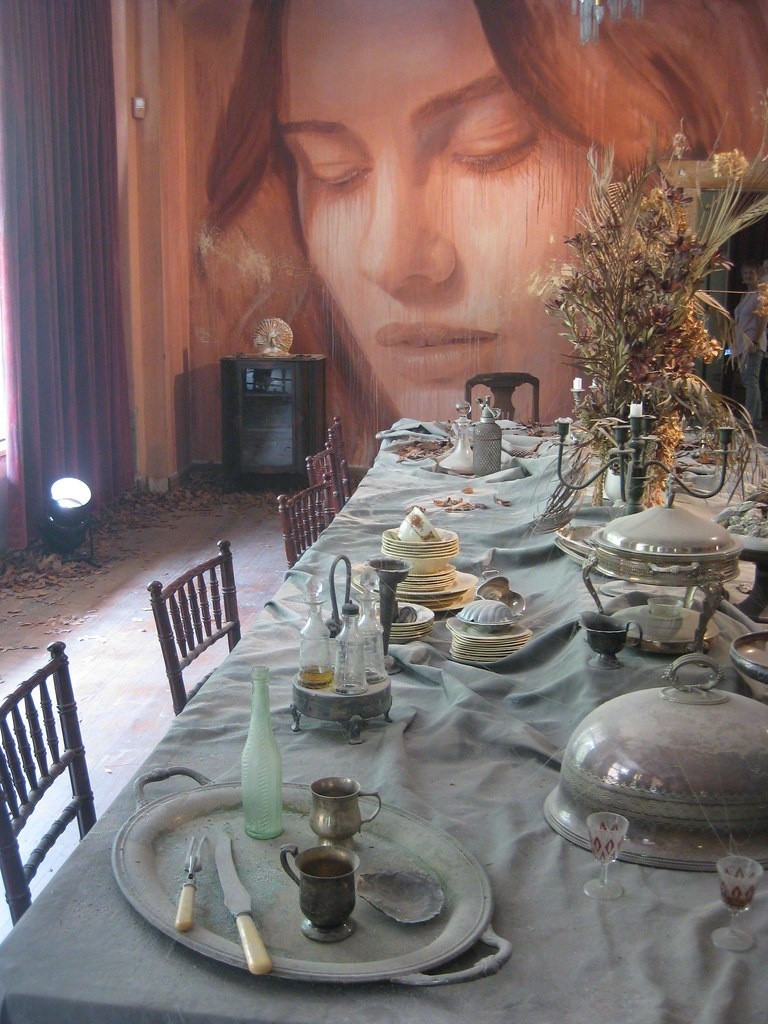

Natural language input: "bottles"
[473,396,501,477]
[241,665,284,839]
[296,575,333,687]
[330,600,369,695]
[356,566,388,684]
[439,400,474,474]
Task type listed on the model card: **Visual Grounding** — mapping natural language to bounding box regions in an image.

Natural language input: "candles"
[630,404,642,417]
[572,377,582,389]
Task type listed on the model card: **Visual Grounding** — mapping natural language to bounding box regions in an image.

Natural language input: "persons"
[729,262,768,434]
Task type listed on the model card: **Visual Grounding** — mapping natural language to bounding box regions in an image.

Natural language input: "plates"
[376,601,435,643]
[554,524,606,571]
[446,617,533,662]
[600,579,687,597]
[350,563,479,611]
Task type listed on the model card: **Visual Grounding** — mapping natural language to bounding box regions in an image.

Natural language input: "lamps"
[31,478,101,569]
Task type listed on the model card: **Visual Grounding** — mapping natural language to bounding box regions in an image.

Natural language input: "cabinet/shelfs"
[221,353,325,492]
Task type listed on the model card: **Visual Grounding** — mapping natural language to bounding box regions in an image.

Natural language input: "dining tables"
[0,422,768,1024]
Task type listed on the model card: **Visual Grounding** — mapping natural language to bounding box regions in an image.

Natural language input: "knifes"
[214,834,272,974]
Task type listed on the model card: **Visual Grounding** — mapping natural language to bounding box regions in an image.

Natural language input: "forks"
[175,836,207,930]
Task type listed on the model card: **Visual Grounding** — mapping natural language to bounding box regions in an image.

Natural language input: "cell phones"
[724,349,731,355]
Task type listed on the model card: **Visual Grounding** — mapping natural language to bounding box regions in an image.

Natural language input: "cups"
[310,777,382,851]
[645,597,685,640]
[280,843,361,941]
[578,610,643,669]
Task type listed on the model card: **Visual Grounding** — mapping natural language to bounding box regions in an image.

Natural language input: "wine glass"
[711,856,763,952]
[583,811,629,901]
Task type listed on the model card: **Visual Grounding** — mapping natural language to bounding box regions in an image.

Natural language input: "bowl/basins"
[455,613,514,634]
[381,506,460,575]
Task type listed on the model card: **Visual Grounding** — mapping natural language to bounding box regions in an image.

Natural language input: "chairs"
[276,472,335,570]
[305,441,341,530]
[147,540,240,715]
[0,641,97,927]
[464,372,539,425]
[328,416,351,506]
